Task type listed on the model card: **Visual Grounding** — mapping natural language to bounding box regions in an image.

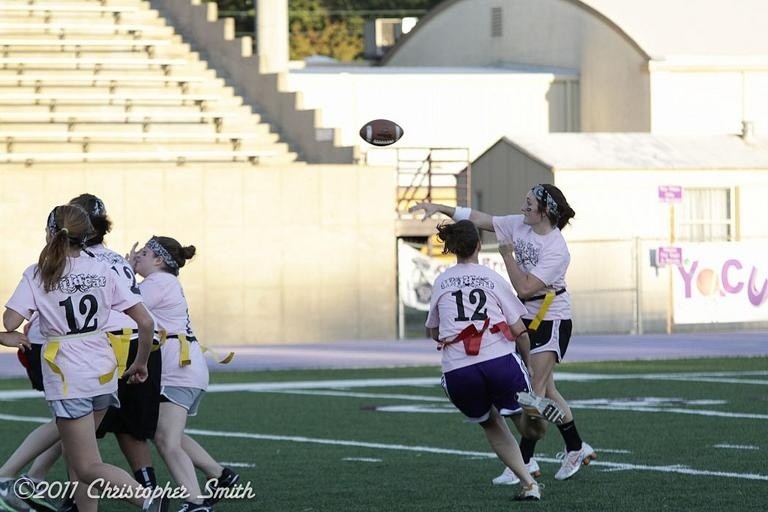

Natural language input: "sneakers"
[555,440,597,480]
[515,482,545,499]
[134,465,238,512]
[516,391,567,424]
[0,474,79,512]
[492,455,542,485]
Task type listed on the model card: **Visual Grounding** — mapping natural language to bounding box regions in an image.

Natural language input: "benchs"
[2,0,299,164]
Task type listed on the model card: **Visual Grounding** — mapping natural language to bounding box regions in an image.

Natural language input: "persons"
[426,219,567,501]
[1,192,239,511]
[408,184,597,486]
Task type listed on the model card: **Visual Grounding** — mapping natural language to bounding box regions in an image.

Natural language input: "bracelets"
[450,205,472,221]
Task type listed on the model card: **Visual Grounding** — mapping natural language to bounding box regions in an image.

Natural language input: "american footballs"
[360,119,405,146]
